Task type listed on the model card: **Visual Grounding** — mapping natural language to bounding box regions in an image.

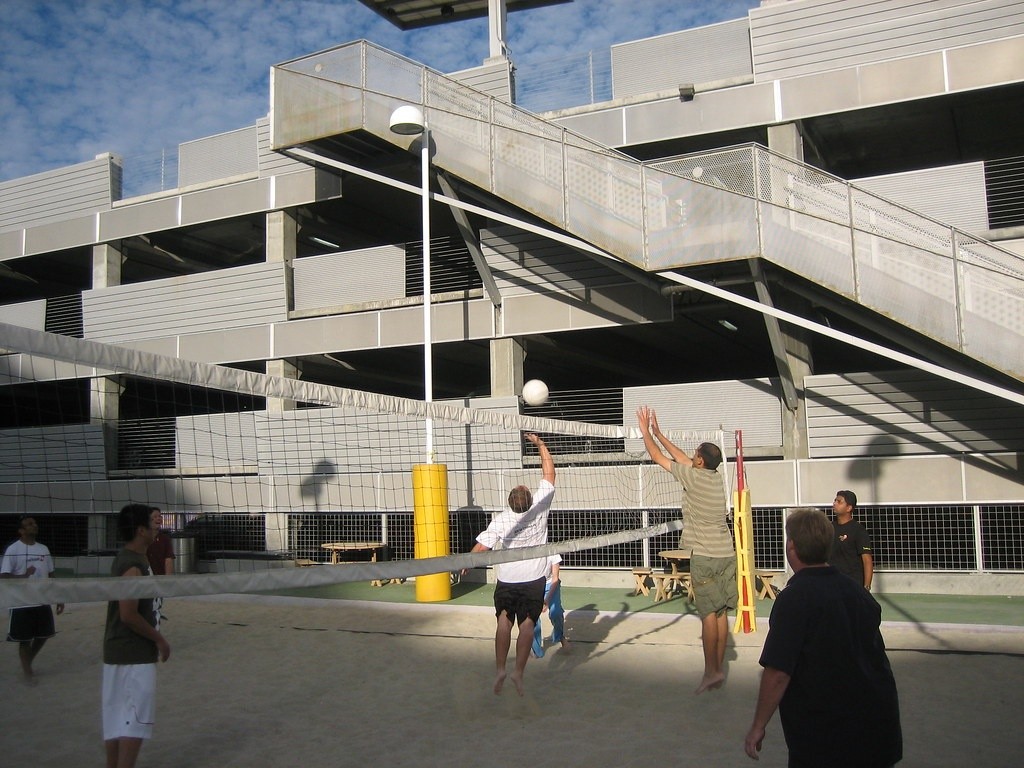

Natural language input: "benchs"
[633,568,777,603]
[295,560,401,586]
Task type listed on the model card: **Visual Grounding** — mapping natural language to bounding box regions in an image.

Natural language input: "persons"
[636,405,739,695]
[744,509,903,768]
[531,553,570,658]
[145,506,176,575]
[826,490,873,591]
[0,514,65,682]
[102,504,171,768]
[462,434,556,698]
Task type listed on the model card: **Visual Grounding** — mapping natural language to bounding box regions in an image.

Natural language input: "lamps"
[679,83,696,100]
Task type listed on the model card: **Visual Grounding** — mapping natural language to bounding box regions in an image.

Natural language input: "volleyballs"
[522,379,549,406]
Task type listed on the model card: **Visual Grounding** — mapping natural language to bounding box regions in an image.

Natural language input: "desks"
[659,550,693,598]
[321,542,386,586]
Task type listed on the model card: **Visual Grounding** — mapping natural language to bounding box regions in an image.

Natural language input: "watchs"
[863,585,871,590]
